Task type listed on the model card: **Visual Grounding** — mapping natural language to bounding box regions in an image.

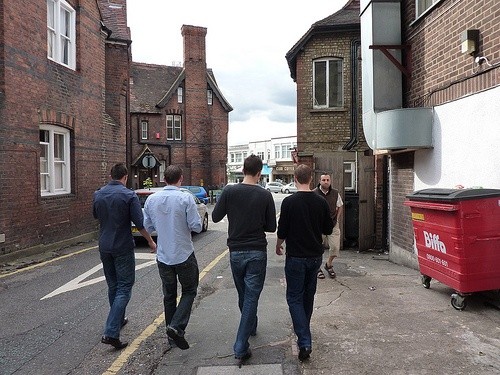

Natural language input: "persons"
[92,164,156,349]
[144,165,202,350]
[276,165,333,359]
[312,172,343,279]
[212,155,277,359]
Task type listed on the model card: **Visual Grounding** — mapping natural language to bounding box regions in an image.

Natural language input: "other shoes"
[298,346,312,363]
[235,348,252,360]
[166,328,190,350]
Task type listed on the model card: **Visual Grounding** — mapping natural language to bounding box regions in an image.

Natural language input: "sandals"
[317,268,325,278]
[324,262,336,278]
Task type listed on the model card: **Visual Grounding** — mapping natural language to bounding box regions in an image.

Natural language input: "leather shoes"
[101,336,128,349]
[121,317,128,328]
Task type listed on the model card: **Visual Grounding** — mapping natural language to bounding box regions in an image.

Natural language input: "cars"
[131,188,209,237]
[282,182,297,194]
[178,185,208,205]
[265,182,284,193]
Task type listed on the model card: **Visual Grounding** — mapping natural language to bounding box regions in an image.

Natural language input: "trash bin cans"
[404,186,499,311]
